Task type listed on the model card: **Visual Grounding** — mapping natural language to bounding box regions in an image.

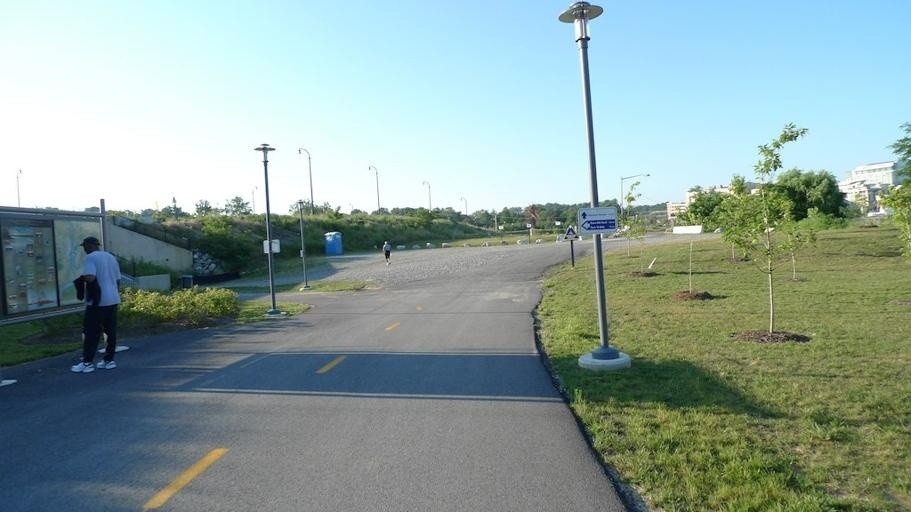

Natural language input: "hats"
[80,237,100,246]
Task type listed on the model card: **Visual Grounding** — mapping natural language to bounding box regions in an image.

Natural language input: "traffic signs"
[577,207,618,236]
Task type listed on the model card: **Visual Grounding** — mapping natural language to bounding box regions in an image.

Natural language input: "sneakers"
[96,359,117,369]
[71,362,95,373]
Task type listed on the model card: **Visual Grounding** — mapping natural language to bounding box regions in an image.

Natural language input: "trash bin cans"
[324,232,344,256]
[182,275,193,288]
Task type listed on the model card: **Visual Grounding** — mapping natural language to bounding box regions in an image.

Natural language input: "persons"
[69,236,123,375]
[382,242,394,265]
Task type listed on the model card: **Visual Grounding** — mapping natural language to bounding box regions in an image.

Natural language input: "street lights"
[369,164,381,209]
[423,181,431,214]
[253,143,277,309]
[558,1,620,359]
[461,197,467,216]
[621,173,650,215]
[298,147,314,215]
[297,199,310,288]
